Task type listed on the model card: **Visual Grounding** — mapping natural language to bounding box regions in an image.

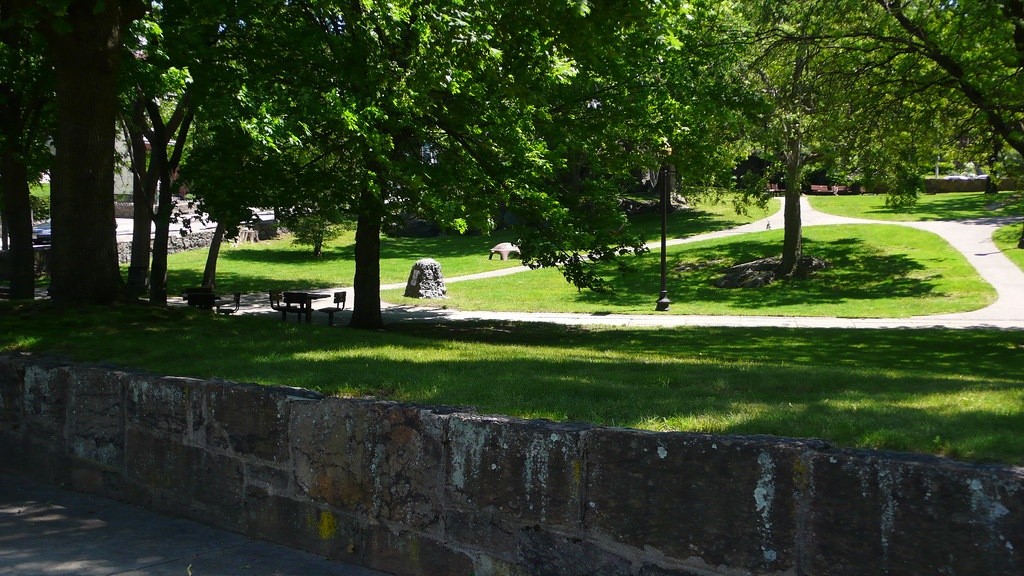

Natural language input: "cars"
[32,221,51,244]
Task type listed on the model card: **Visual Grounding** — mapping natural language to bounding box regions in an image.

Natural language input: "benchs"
[318,291,346,326]
[269,289,297,319]
[283,291,314,321]
[183,286,241,315]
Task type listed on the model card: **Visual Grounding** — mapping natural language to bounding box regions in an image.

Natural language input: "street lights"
[656,141,672,310]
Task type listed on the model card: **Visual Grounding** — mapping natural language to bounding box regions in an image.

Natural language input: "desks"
[286,293,331,321]
[182,294,221,309]
